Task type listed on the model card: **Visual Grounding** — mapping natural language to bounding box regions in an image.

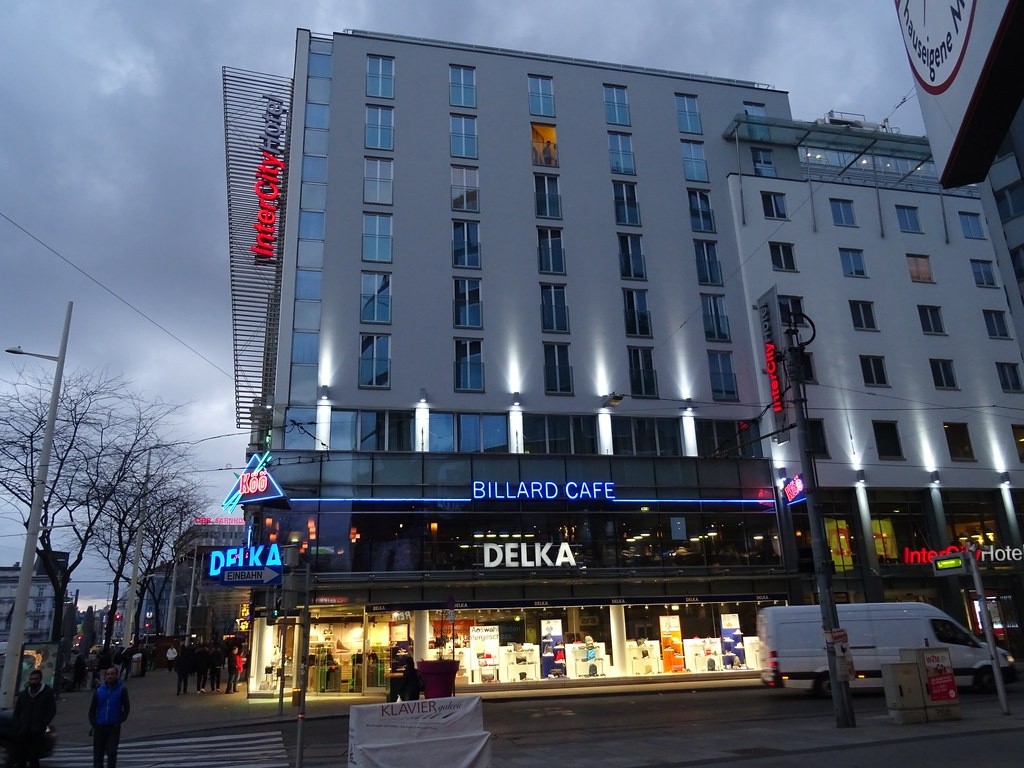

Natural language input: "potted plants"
[418,636,460,699]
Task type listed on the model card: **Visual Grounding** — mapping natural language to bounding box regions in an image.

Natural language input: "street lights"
[178,553,197,648]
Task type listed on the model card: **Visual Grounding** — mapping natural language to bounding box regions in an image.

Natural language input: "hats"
[92,664,101,667]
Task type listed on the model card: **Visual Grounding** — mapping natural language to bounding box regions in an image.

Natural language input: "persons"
[324,649,334,665]
[399,660,420,701]
[167,644,177,672]
[53,655,89,700]
[368,650,378,663]
[175,636,247,696]
[88,666,130,768]
[13,670,56,768]
[112,643,161,681]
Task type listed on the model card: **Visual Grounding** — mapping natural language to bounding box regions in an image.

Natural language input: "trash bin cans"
[131,653,143,677]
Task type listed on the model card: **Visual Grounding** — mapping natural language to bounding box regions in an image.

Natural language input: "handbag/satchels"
[89,727,95,736]
[413,669,425,692]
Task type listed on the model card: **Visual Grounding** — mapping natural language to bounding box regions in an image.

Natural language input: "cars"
[89,644,105,654]
[0,706,57,768]
[0,641,8,668]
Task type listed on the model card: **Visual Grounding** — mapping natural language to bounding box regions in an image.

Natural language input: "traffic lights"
[274,597,281,616]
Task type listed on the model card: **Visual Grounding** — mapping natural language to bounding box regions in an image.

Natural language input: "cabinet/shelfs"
[574,648,604,677]
[436,654,469,686]
[752,641,760,669]
[506,651,537,680]
[308,635,353,680]
[629,647,659,675]
[479,656,499,683]
[370,645,391,675]
[542,639,566,663]
[722,633,744,657]
[693,644,719,671]
[266,662,293,687]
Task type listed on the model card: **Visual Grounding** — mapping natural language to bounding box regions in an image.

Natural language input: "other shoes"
[200,688,206,693]
[233,690,239,692]
[226,690,234,693]
[196,691,201,694]
[216,689,222,692]
[176,687,181,696]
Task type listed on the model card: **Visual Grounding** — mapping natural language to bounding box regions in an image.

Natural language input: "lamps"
[1001,472,1010,485]
[321,385,328,400]
[930,471,940,483]
[514,392,520,406]
[600,395,607,407]
[970,531,981,538]
[266,395,273,410]
[986,528,994,535]
[959,532,968,540]
[418,388,426,402]
[685,398,692,412]
[602,392,623,407]
[777,468,787,481]
[857,470,865,483]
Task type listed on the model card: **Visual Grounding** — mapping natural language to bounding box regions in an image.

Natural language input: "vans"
[759,601,1019,700]
